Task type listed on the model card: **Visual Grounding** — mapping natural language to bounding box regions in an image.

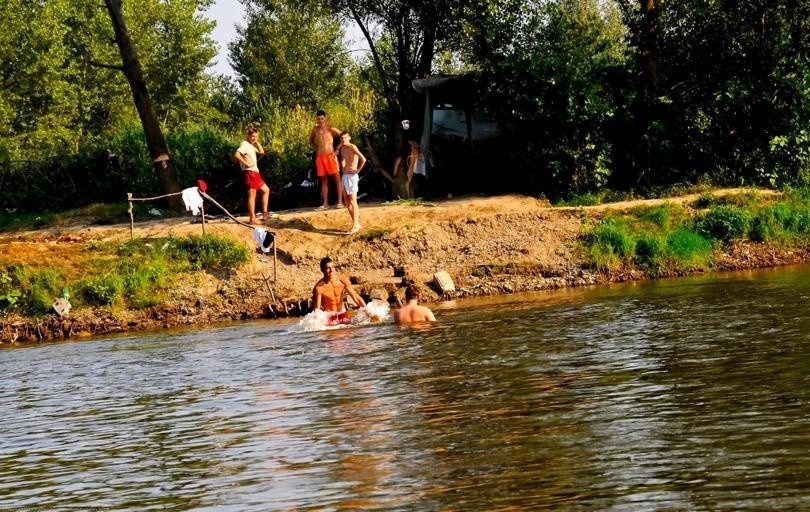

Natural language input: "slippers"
[335,202,344,208]
[314,205,330,212]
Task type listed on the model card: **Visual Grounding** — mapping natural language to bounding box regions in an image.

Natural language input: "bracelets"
[336,147,340,150]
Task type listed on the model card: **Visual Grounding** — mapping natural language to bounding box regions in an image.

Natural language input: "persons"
[310,257,366,324]
[234,128,271,225]
[393,284,437,324]
[359,137,393,184]
[309,109,343,211]
[393,146,407,193]
[407,137,423,202]
[339,132,368,234]
